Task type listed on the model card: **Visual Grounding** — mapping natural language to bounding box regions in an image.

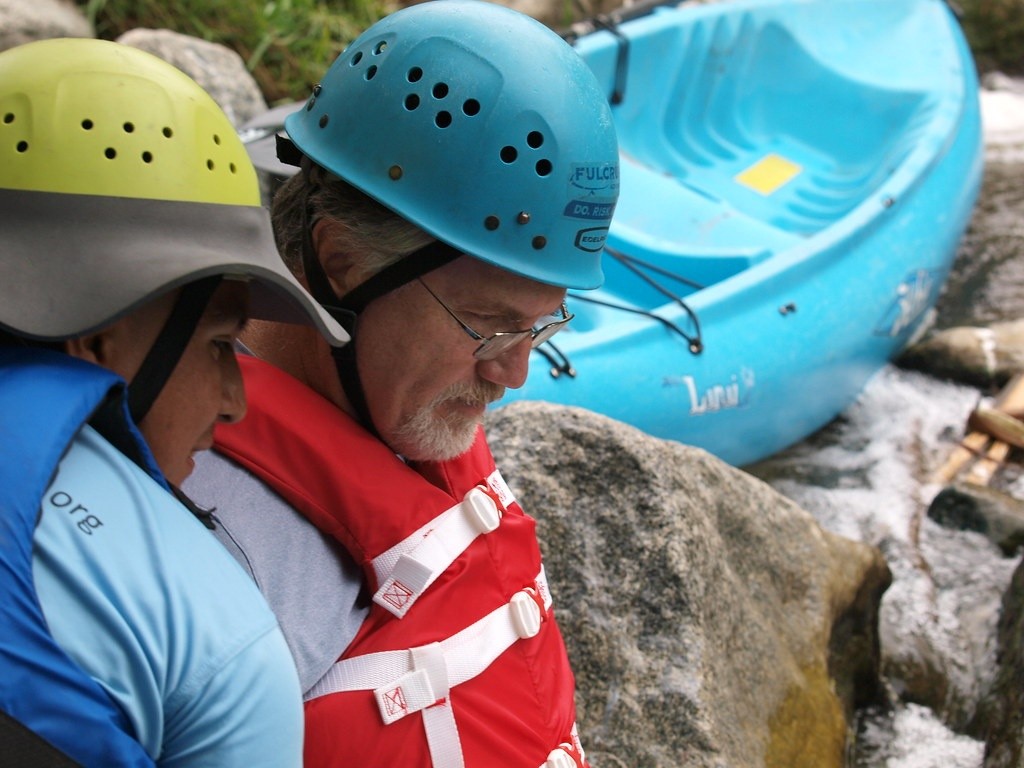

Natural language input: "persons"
[1,37,357,768]
[182,0,624,765]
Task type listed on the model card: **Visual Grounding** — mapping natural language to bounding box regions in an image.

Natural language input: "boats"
[480,1,983,478]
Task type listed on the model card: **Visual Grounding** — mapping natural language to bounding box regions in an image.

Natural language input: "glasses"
[417,276,575,361]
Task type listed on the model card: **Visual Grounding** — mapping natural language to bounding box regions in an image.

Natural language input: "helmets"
[284,0,621,290]
[1,38,353,347]
